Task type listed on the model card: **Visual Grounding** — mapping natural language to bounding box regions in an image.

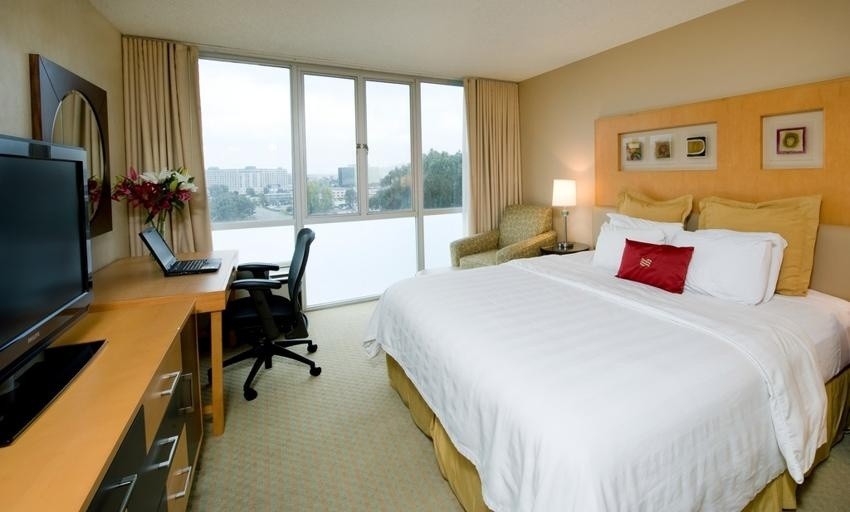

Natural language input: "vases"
[144,217,166,255]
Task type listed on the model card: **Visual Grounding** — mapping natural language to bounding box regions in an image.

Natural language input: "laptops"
[138,228,221,277]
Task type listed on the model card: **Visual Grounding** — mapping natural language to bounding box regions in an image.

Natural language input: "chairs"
[203,225,324,400]
[447,202,557,270]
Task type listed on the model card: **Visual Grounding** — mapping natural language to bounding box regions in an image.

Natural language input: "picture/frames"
[775,126,806,156]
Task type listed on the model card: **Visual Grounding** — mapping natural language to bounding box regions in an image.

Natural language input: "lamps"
[550,176,582,251]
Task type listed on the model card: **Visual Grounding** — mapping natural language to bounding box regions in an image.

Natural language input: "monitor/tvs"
[0,133,107,447]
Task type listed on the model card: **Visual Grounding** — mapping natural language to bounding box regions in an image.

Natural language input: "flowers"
[86,172,102,206]
[111,162,199,235]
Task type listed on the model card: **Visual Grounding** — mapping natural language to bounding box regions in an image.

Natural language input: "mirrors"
[26,47,114,242]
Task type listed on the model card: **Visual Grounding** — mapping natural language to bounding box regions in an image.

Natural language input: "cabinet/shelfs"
[1,298,211,512]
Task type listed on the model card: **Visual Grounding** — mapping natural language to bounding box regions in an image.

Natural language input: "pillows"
[696,230,786,304]
[592,221,665,270]
[619,188,695,227]
[616,237,696,296]
[672,230,774,307]
[606,212,684,242]
[694,193,824,298]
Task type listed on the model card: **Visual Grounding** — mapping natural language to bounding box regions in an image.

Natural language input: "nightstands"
[540,240,591,255]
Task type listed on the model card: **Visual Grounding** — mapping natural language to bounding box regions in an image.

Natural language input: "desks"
[85,246,239,437]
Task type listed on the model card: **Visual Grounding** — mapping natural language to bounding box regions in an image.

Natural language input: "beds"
[366,191,850,512]
